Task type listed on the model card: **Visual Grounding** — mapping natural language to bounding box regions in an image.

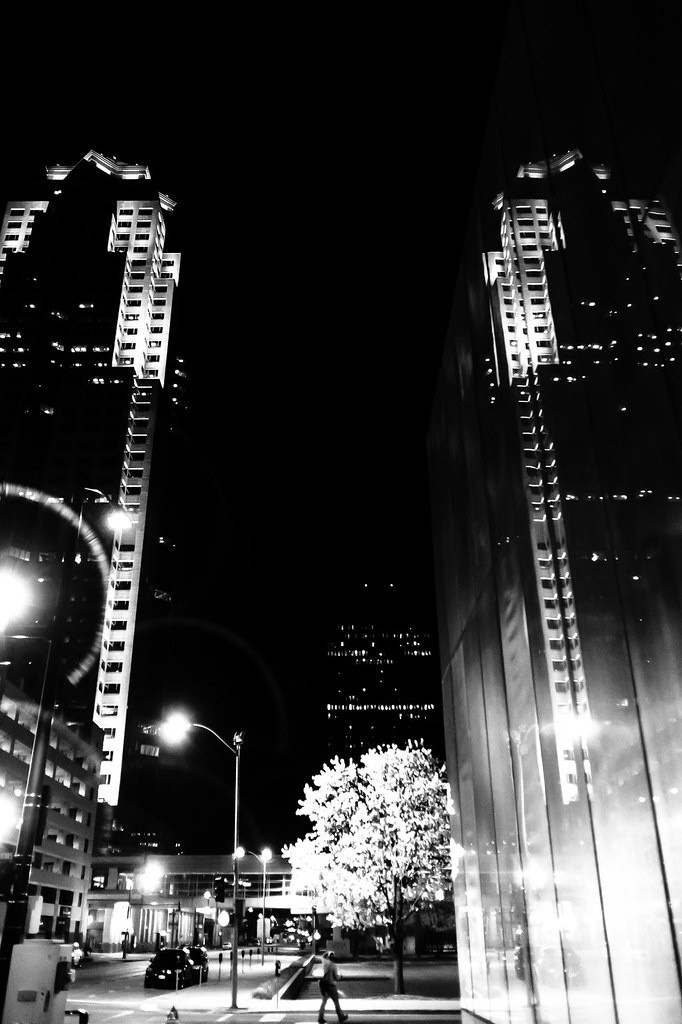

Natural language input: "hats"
[327,951,335,958]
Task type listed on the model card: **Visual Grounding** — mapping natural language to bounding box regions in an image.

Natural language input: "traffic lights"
[214,877,224,902]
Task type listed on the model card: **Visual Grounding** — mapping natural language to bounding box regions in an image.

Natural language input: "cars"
[145,948,193,989]
[222,942,232,950]
[298,938,311,947]
[176,946,209,983]
[248,939,260,946]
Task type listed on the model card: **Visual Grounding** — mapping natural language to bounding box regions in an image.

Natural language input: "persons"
[317,951,348,1024]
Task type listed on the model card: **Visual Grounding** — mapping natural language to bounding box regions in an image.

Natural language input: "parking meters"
[219,953,222,979]
[200,954,204,986]
[176,954,181,991]
[257,948,260,965]
[242,950,244,973]
[230,951,232,978]
[275,960,281,1008]
[249,949,252,967]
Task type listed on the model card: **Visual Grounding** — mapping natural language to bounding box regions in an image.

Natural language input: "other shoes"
[318,1018,327,1024]
[339,1014,348,1022]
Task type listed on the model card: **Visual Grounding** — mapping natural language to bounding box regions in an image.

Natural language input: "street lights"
[0,488,131,1024]
[169,712,244,1009]
[237,847,272,965]
